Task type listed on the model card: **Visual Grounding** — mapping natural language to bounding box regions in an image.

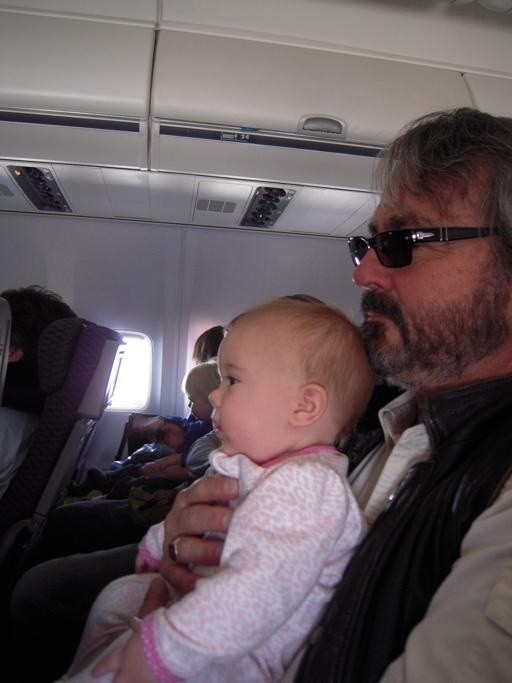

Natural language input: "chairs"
[0,315,120,564]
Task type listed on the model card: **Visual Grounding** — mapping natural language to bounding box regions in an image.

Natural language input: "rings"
[168,535,180,562]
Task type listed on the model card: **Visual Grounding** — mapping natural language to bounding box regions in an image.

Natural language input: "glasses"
[347,226,498,268]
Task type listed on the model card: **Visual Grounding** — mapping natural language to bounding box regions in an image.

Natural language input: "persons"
[78,320,227,500]
[62,298,371,682]
[0,282,78,502]
[0,292,324,683]
[135,111,512,680]
